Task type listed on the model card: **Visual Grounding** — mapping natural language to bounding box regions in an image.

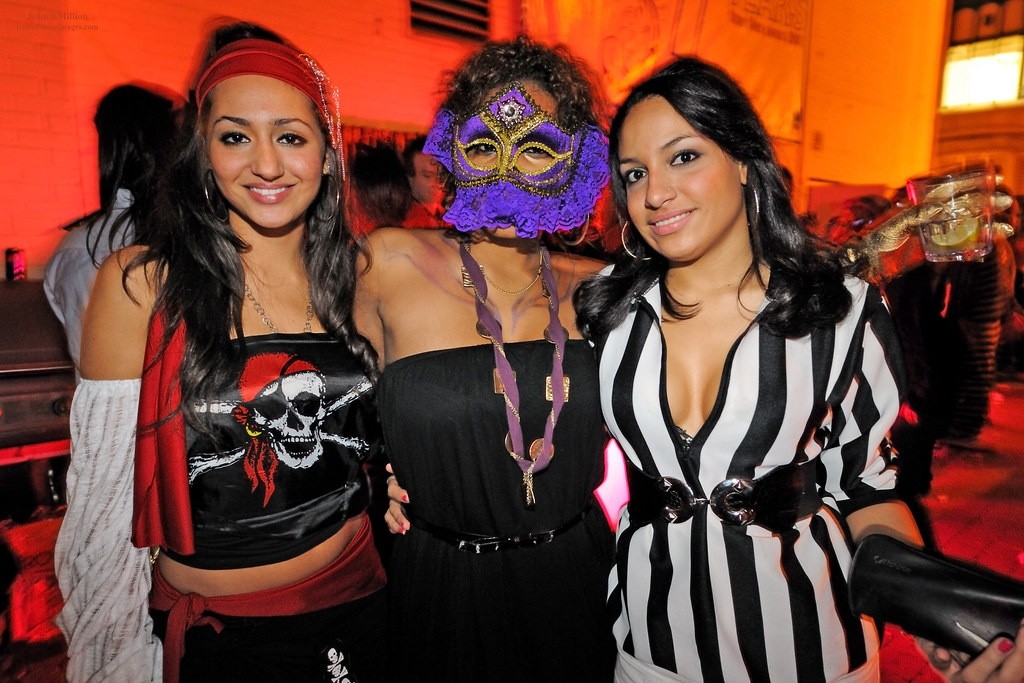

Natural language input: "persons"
[44,20,1024,683]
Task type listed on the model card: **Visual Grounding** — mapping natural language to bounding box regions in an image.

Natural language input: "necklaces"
[245,279,315,332]
[458,232,568,506]
[464,241,542,294]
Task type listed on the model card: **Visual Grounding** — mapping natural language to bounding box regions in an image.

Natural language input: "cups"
[905,153,992,264]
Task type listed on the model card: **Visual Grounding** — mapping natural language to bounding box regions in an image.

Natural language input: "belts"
[627,463,819,528]
[411,513,587,553]
